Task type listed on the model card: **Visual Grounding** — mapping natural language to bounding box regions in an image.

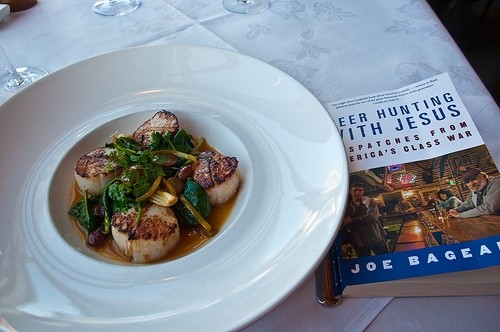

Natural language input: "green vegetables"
[66,128,211,235]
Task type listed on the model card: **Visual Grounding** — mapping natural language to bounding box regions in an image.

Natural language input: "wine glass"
[92,0,141,16]
[0,44,49,98]
[222,0,270,14]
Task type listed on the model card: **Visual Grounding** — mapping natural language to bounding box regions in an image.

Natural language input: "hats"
[462,168,482,184]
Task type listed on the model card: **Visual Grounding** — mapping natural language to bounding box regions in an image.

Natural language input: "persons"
[345,182,390,258]
[437,189,461,210]
[447,168,500,218]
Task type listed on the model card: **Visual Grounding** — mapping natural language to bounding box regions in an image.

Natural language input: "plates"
[0,46,348,332]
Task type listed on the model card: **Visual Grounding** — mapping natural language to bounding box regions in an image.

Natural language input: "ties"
[474,190,483,206]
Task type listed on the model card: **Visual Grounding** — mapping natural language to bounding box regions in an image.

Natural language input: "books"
[324,72,500,298]
